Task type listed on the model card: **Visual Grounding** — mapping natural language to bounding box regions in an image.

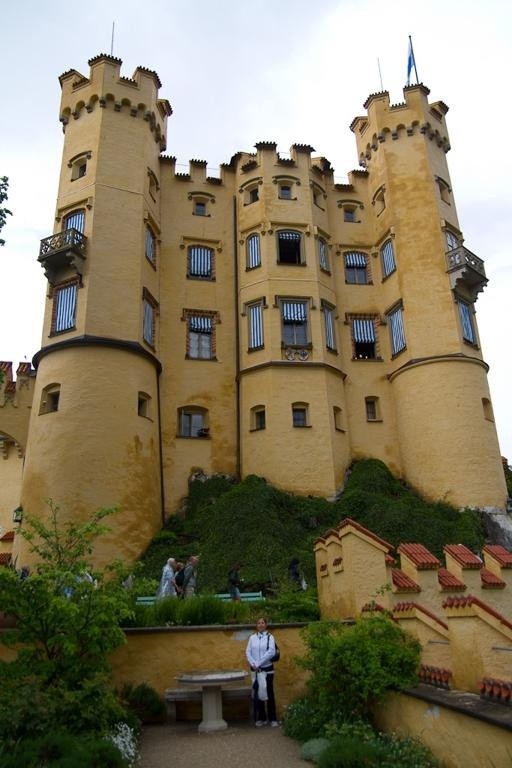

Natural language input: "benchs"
[164,685,256,726]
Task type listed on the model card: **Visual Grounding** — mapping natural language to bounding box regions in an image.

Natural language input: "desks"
[175,670,250,734]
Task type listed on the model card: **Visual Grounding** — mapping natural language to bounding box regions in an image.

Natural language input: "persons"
[245,616,282,728]
[176,556,197,599]
[286,556,305,592]
[157,557,182,600]
[228,564,242,603]
[174,562,183,574]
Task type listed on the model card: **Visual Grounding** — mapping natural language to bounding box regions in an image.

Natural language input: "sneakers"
[255,720,279,727]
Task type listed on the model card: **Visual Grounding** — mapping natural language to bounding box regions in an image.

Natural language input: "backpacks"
[175,564,191,586]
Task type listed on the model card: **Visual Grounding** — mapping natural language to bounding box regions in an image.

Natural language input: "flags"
[406,40,415,85]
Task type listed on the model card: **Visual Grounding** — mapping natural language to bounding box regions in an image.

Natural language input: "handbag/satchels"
[267,648,280,662]
